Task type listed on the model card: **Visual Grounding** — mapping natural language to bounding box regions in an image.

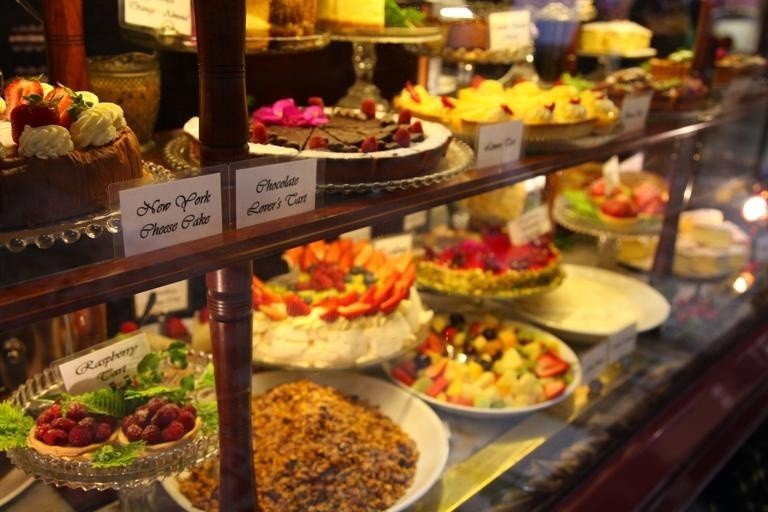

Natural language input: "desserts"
[186,45,768,233]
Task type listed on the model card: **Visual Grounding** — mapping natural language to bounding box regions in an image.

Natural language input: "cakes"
[0,78,152,230]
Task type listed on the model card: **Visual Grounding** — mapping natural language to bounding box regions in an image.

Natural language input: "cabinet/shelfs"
[0,0,766,512]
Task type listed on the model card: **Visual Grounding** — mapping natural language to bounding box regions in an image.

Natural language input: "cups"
[88,56,162,155]
[533,16,577,85]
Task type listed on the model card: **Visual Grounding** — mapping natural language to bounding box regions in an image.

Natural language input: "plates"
[601,94,708,114]
[130,32,334,58]
[510,262,672,341]
[3,156,183,258]
[455,114,598,141]
[160,369,451,511]
[381,311,584,419]
[1,341,220,492]
[160,125,481,197]
[441,41,534,67]
